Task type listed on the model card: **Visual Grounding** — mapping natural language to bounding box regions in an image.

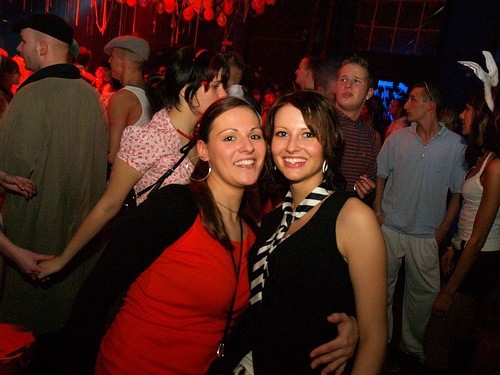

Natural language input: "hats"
[20,11,73,46]
[104,36,150,62]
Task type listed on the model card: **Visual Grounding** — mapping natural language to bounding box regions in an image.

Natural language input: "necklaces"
[218,212,243,359]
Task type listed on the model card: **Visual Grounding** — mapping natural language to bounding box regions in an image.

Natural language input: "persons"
[247,89,388,375]
[63,97,359,375]
[222,49,339,225]
[336,50,500,375]
[0,14,230,375]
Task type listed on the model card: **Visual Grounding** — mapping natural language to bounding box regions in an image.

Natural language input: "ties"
[248,180,335,310]
